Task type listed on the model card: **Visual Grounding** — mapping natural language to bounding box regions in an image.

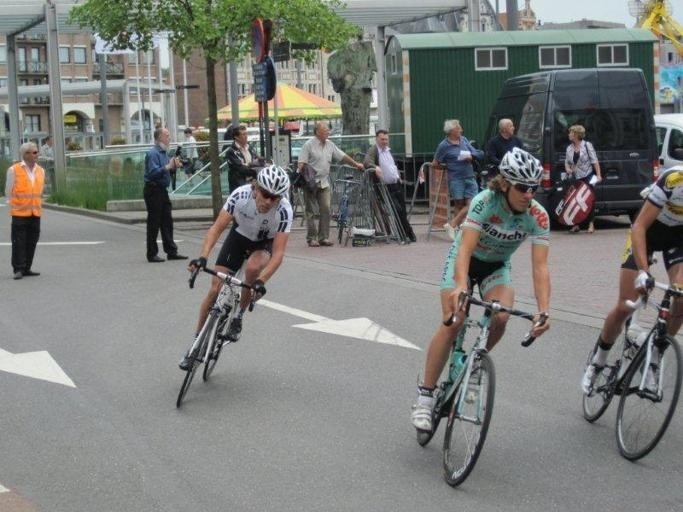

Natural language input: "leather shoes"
[23,270,40,276]
[152,255,165,262]
[167,254,188,260]
[14,272,22,280]
[407,230,415,242]
[320,240,333,246]
[309,239,319,247]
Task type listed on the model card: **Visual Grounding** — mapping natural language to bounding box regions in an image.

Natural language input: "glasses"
[26,151,37,154]
[258,188,282,202]
[504,179,538,193]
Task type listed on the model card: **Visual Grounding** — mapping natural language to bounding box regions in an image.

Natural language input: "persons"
[179,164,293,370]
[296,124,363,247]
[183,129,196,185]
[412,147,551,432]
[5,141,45,279]
[41,135,54,192]
[433,120,484,240]
[488,118,525,176]
[565,124,602,233]
[225,126,266,194]
[363,130,416,243]
[143,128,188,262]
[582,166,682,397]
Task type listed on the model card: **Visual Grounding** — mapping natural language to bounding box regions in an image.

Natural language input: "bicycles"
[176,260,267,408]
[416,290,549,487]
[582,277,683,460]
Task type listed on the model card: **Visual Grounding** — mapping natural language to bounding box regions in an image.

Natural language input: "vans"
[483,69,661,227]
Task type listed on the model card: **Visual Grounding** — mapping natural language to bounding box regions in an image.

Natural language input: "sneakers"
[464,370,481,405]
[582,363,603,398]
[443,223,455,242]
[638,360,657,394]
[411,394,434,432]
[179,349,201,371]
[225,317,241,343]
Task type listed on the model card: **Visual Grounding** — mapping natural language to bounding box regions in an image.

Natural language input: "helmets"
[500,147,543,188]
[257,165,291,196]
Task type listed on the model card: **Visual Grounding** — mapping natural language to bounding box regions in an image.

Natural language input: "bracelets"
[352,160,356,166]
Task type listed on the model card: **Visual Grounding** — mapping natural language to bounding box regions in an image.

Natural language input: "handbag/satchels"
[590,161,604,181]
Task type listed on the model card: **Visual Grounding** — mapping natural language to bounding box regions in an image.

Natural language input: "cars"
[655,113,683,169]
